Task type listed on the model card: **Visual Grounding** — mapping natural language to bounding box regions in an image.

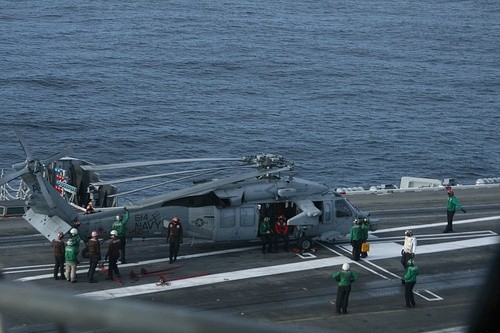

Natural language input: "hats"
[92,231,99,237]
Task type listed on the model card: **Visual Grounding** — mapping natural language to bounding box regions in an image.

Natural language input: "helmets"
[58,231,63,238]
[408,259,415,266]
[405,229,413,236]
[361,216,369,223]
[172,217,180,222]
[263,217,270,222]
[354,217,361,223]
[342,263,350,270]
[116,214,123,220]
[111,230,118,237]
[70,228,78,236]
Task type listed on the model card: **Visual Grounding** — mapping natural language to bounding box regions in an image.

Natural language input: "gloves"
[411,253,414,258]
[180,241,183,244]
[401,250,405,255]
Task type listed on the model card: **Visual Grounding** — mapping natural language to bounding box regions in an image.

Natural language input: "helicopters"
[0,125,380,262]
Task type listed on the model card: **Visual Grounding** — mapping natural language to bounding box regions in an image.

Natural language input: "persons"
[165,215,186,262]
[349,214,375,258]
[48,206,131,281]
[400,256,419,304]
[445,189,468,233]
[397,227,419,271]
[333,262,360,312]
[257,215,293,251]
